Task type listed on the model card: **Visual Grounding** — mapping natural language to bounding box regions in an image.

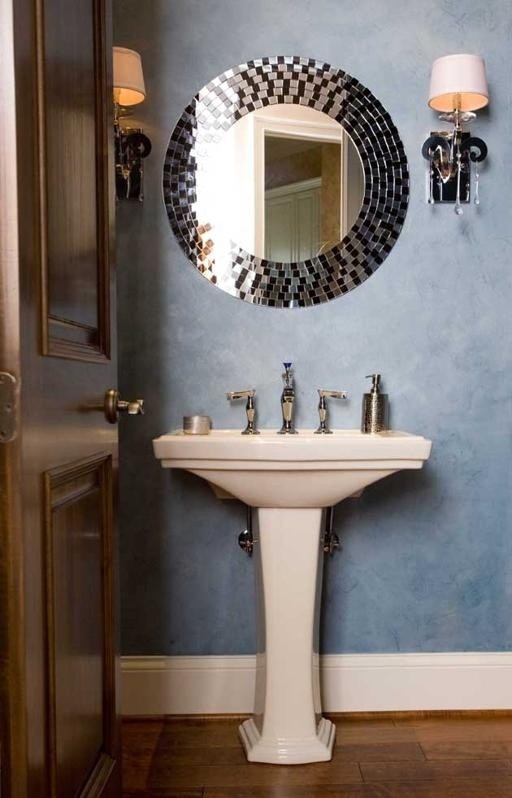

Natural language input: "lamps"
[422,54,490,214]
[111,46,153,204]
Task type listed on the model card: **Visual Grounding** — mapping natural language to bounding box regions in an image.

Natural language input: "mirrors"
[163,54,410,310]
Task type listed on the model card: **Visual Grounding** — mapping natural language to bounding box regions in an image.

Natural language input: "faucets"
[276,361,300,435]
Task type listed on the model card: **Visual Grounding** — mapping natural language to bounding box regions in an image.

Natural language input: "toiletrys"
[183,416,210,434]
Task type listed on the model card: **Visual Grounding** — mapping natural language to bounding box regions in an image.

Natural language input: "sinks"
[153,425,433,510]
